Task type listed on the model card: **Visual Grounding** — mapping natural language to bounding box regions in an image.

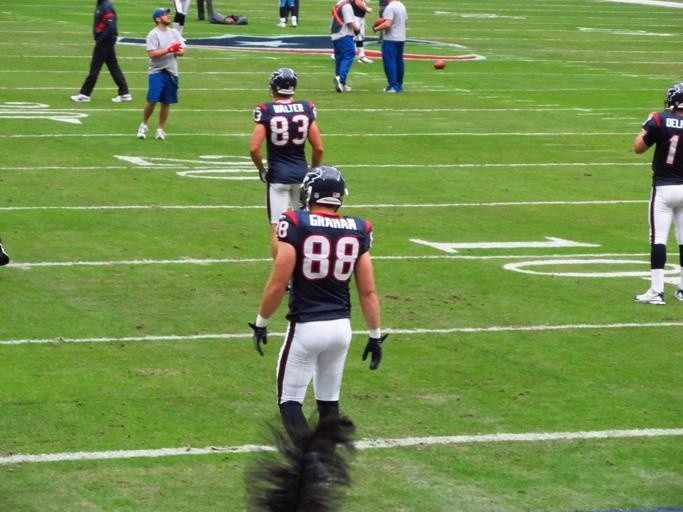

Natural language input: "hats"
[152,7,170,22]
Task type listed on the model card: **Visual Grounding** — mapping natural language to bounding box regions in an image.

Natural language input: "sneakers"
[70,94,91,102]
[0,244,9,266]
[334,75,403,93]
[276,22,287,27]
[673,289,683,301]
[136,122,148,139]
[331,54,336,60]
[291,21,297,27]
[155,128,165,140]
[357,56,373,64]
[633,288,666,305]
[112,94,132,103]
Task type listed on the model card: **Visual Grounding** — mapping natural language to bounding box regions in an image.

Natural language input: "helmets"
[665,82,682,113]
[299,166,344,212]
[268,68,298,97]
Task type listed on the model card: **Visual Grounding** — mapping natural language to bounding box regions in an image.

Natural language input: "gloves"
[362,333,389,370]
[259,168,268,183]
[353,34,362,41]
[247,322,267,357]
[167,41,183,52]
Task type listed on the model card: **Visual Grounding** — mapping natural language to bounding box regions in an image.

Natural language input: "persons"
[634,82,683,304]
[248,67,324,291]
[277,0,297,27]
[249,165,388,444]
[329,0,407,92]
[137,8,186,140]
[71,0,132,103]
[174,0,190,26]
[197,0,212,20]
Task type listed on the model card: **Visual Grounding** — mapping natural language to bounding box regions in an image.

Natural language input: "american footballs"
[433,61,445,69]
